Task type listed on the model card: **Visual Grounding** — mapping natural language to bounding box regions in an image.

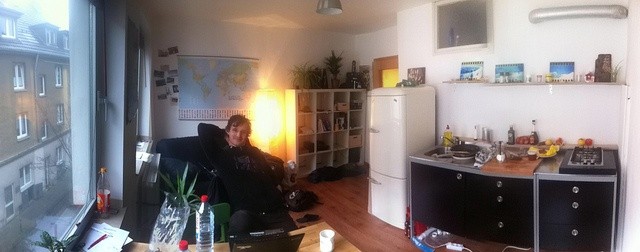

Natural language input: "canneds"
[545,72,554,84]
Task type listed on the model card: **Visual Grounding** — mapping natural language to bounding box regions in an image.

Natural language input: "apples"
[585,138,592,146]
[578,138,585,146]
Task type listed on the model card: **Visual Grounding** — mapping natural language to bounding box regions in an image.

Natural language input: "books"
[299,126,314,133]
[334,113,349,131]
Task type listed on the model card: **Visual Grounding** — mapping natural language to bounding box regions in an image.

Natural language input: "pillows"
[164,147,216,185]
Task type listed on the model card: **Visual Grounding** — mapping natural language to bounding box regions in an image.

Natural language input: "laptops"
[227,227,305,252]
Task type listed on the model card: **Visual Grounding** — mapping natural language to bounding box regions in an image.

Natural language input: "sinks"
[423,144,482,169]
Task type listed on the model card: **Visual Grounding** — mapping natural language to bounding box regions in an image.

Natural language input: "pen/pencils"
[87,234,106,250]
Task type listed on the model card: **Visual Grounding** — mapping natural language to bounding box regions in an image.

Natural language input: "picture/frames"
[126,16,140,123]
[429,0,492,58]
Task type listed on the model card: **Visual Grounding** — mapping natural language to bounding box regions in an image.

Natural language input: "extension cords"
[447,242,464,251]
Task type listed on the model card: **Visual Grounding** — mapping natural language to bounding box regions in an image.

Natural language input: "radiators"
[140,152,161,213]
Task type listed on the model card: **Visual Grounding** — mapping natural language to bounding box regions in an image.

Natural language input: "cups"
[320,230,336,252]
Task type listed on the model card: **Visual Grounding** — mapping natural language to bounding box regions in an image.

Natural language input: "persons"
[197,115,299,242]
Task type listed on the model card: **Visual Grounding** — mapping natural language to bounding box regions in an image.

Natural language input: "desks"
[114,221,361,251]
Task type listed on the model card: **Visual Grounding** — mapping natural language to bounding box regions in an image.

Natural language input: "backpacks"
[285,189,317,211]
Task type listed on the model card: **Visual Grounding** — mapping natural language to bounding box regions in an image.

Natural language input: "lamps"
[316,0,342,17]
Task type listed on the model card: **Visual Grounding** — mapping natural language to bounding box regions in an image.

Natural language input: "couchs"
[156,134,284,208]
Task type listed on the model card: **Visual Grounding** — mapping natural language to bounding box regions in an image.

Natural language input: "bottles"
[508,126,515,145]
[175,240,192,252]
[196,195,215,252]
[96,168,111,216]
[528,120,538,144]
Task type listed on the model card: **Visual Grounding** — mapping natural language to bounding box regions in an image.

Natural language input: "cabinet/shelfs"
[444,78,628,87]
[406,157,534,251]
[535,173,618,251]
[284,88,367,179]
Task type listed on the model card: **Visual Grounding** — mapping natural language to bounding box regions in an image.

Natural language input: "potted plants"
[610,59,624,82]
[321,48,344,88]
[288,59,321,89]
[150,162,205,252]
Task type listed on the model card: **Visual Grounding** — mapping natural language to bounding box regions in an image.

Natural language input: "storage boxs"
[349,134,362,148]
[351,99,362,109]
[335,100,350,111]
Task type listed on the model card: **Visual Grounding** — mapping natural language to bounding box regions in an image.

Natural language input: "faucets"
[441,135,459,146]
